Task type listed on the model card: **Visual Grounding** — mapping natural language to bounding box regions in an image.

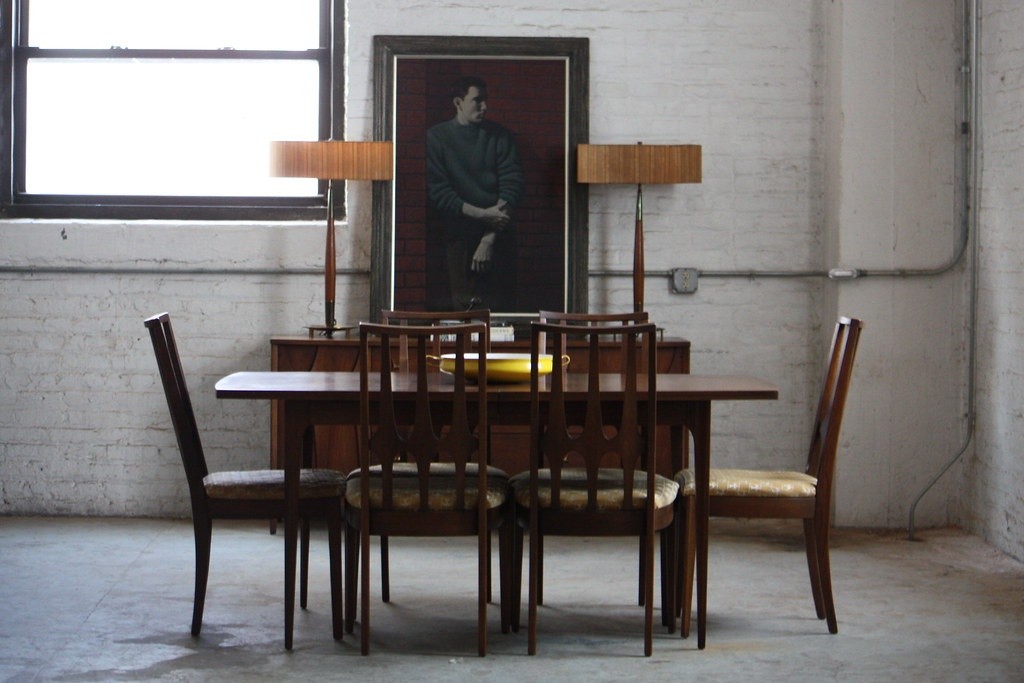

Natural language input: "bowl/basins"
[426,351,570,380]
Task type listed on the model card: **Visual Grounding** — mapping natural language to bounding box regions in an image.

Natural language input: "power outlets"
[673,268,699,294]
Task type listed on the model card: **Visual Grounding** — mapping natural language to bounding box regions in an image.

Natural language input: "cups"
[439,320,515,343]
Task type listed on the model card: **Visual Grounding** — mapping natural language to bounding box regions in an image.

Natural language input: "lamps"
[266,138,393,338]
[578,141,702,332]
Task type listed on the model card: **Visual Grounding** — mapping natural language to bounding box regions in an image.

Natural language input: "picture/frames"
[371,34,590,336]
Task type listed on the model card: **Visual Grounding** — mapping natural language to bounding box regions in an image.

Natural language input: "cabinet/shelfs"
[269,334,690,535]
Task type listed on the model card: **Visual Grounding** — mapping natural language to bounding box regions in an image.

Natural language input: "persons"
[426,77,524,312]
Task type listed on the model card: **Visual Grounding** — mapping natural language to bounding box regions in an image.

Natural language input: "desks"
[215,371,779,649]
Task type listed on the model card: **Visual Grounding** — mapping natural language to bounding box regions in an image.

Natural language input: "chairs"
[378,308,492,602]
[538,309,649,608]
[146,311,356,641]
[662,314,863,634]
[527,321,657,657]
[346,322,510,655]
